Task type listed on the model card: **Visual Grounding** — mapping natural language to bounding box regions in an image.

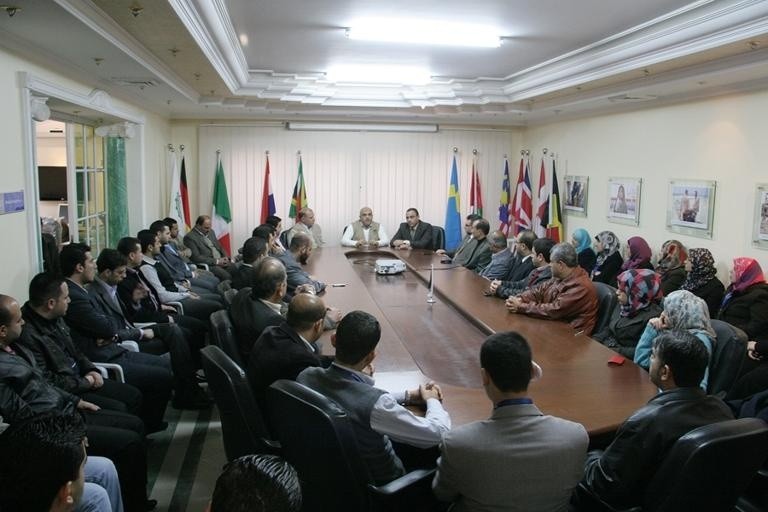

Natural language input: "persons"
[613,186,627,214]
[1,207,452,508]
[680,189,690,222]
[690,190,700,222]
[431,214,768,512]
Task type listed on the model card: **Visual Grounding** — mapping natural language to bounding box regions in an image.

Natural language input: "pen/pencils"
[575,330,583,336]
[333,284,345,287]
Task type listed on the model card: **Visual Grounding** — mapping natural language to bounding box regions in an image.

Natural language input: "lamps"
[284,122,439,133]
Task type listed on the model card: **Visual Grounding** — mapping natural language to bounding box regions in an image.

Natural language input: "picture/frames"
[751,183,768,252]
[665,179,716,239]
[606,178,642,227]
[562,175,589,218]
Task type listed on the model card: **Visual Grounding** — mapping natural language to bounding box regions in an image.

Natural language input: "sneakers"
[175,395,213,410]
[149,421,170,432]
[135,497,159,511]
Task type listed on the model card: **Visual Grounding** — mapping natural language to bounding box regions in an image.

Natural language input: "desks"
[303,246,661,446]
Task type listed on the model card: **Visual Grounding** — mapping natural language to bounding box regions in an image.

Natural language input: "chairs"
[209,308,243,363]
[199,344,258,470]
[430,226,445,251]
[591,281,619,333]
[708,316,748,394]
[645,417,768,512]
[218,279,236,309]
[266,380,436,511]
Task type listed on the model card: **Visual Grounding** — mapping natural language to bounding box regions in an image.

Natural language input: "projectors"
[375,259,406,274]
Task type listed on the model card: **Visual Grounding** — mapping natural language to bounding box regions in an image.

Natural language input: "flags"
[548,162,563,244]
[510,158,523,236]
[444,157,462,251]
[180,156,191,229]
[536,160,549,240]
[289,158,308,218]
[260,158,276,225]
[211,159,232,256]
[516,166,533,234]
[469,162,482,218]
[499,161,511,237]
[169,154,185,237]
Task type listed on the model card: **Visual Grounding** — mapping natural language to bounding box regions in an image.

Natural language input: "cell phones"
[481,288,491,296]
[608,356,624,366]
